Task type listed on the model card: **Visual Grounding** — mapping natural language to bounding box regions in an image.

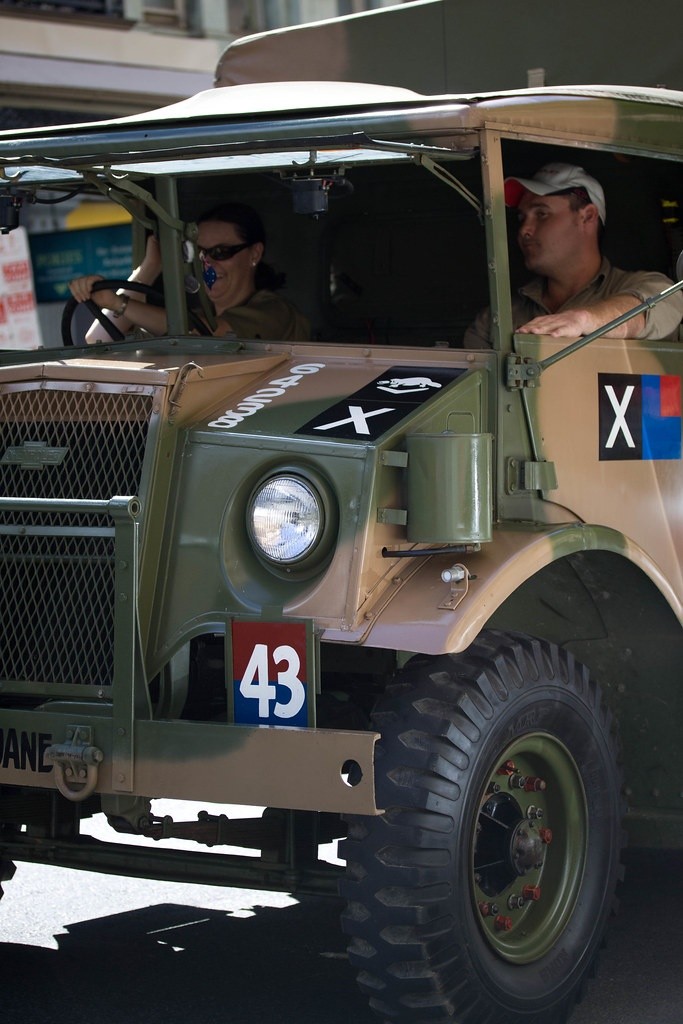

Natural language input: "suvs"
[0,1,683,1023]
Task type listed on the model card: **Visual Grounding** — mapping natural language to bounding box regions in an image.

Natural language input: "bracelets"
[113,294,130,319]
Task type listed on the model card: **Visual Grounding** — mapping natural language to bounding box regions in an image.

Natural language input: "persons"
[66,199,310,344]
[465,164,683,350]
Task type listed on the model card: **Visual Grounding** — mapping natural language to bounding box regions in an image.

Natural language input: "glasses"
[191,241,255,262]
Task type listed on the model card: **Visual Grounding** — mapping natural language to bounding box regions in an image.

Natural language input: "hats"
[503,162,606,225]
